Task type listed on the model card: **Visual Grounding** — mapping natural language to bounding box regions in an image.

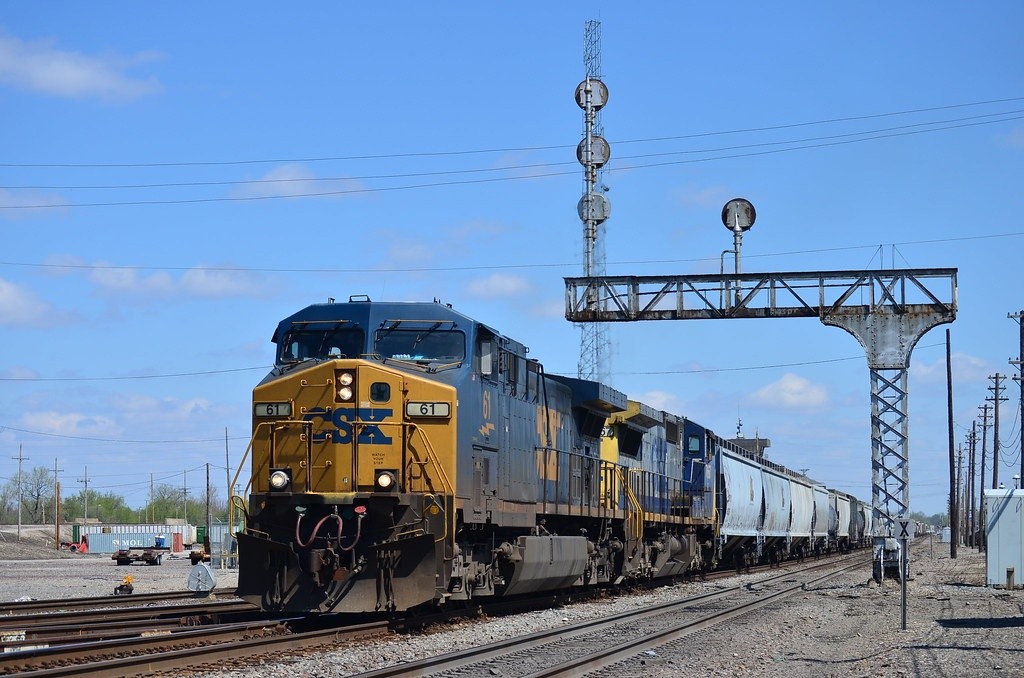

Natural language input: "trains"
[225,295,937,614]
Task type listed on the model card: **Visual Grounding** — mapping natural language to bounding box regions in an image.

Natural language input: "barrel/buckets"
[155,536,165,547]
